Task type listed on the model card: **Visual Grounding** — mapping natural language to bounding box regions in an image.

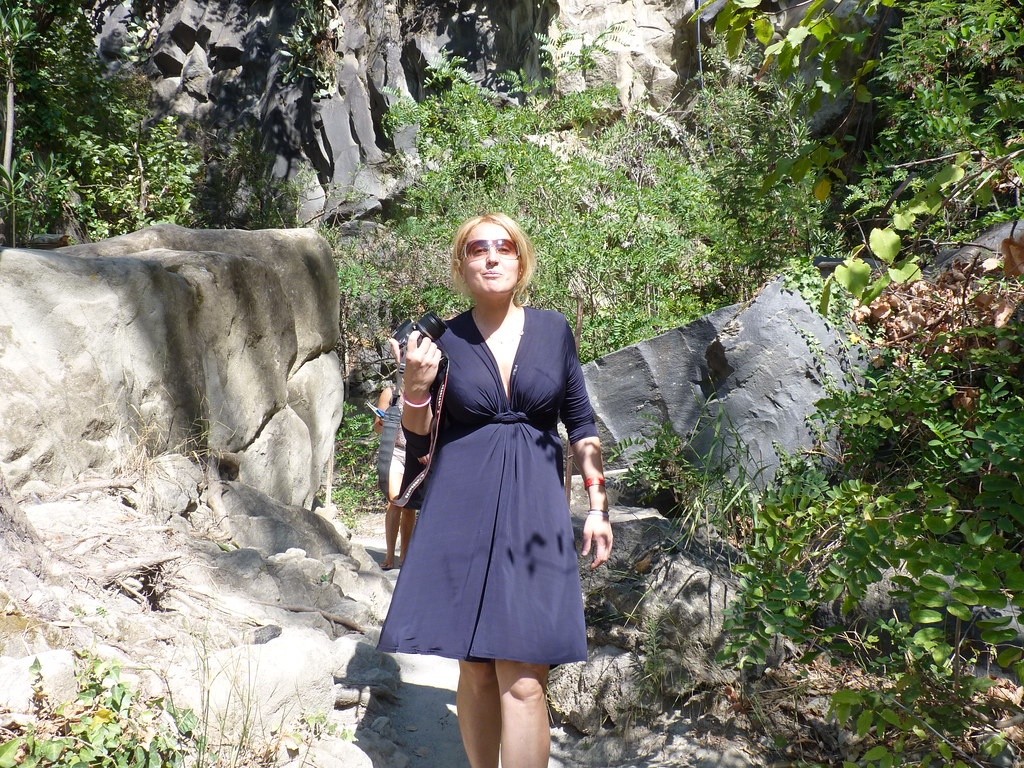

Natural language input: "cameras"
[391,311,450,375]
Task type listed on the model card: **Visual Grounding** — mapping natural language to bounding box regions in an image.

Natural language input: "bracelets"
[403,392,431,408]
[588,509,609,518]
[585,477,605,491]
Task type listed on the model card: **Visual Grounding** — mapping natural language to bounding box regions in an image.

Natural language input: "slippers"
[381,562,394,570]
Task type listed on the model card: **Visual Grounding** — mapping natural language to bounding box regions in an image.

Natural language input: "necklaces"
[474,307,487,336]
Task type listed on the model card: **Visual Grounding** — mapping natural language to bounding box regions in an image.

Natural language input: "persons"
[375,212,613,768]
[374,363,416,570]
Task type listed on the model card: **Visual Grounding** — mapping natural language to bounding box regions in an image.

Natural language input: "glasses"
[467,239,520,260]
